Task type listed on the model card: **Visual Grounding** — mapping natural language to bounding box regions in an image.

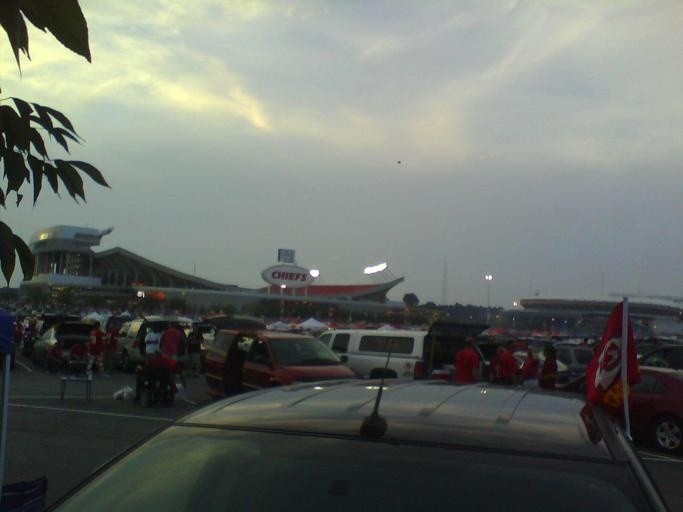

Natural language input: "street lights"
[485,274,493,308]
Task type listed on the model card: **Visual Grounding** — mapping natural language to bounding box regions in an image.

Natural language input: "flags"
[586,302,642,409]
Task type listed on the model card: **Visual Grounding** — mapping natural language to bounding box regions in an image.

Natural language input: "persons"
[455,332,600,391]
[0,313,203,407]
